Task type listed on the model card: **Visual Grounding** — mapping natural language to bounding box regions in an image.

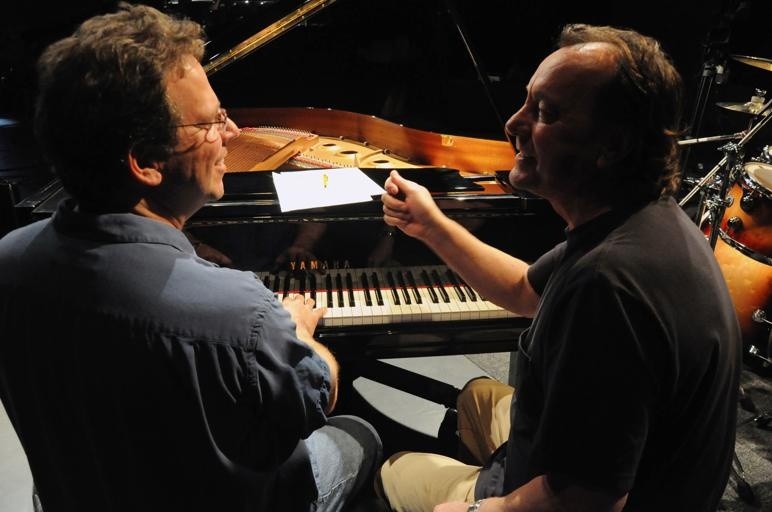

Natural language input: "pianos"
[11,107,566,412]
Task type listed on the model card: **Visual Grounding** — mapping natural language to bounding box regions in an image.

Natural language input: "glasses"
[157,106,230,137]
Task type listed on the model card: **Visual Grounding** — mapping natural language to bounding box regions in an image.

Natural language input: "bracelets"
[465,499,483,512]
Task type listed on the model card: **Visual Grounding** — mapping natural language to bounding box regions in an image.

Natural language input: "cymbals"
[732,55,772,71]
[716,102,772,116]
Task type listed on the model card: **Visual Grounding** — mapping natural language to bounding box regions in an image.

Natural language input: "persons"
[371,16,745,512]
[0,0,386,512]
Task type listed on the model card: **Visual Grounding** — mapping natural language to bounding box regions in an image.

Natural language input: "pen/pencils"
[324,174,328,189]
[464,177,496,181]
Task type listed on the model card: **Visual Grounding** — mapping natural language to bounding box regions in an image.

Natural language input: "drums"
[704,161,771,354]
